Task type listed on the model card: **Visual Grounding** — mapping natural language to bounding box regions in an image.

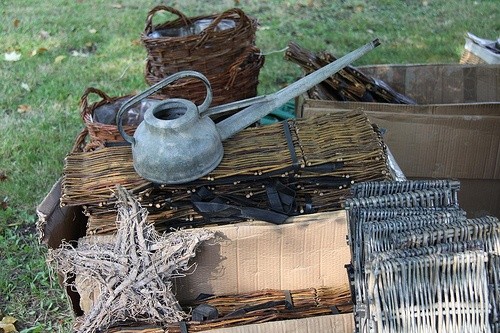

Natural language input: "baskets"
[79,85,170,149]
[138,46,264,112]
[139,2,258,81]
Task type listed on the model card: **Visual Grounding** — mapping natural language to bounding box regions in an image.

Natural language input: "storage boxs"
[294,59,500,221]
[34,142,407,333]
